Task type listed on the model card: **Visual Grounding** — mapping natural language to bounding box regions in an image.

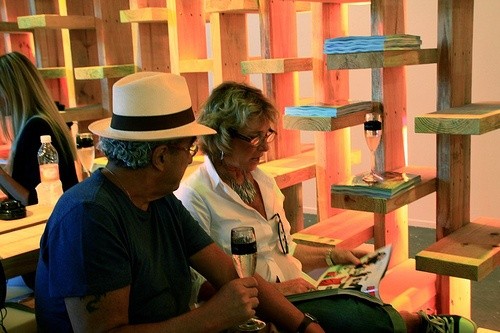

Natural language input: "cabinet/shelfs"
[0,0,500,333]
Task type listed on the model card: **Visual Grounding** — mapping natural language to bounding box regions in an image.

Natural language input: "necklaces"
[217,170,256,204]
[103,166,132,201]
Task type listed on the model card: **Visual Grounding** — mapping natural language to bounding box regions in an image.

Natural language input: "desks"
[0,202,63,279]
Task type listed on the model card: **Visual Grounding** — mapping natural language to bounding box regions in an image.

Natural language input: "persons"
[0,51,79,205]
[172,81,477,333]
[35,72,325,333]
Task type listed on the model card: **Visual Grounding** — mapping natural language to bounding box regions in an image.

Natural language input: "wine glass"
[230,226,266,331]
[75,133,95,177]
[362,112,384,183]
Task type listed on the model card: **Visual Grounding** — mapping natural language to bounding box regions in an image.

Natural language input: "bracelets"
[326,248,334,266]
[296,312,317,333]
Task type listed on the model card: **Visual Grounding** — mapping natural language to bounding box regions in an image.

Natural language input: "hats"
[87,71,217,141]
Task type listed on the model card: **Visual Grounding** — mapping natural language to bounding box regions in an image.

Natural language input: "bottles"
[37,135,59,207]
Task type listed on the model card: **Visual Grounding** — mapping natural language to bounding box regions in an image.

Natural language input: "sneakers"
[416,308,478,333]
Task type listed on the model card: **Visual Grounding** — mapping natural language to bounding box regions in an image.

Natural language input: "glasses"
[233,127,278,146]
[270,212,289,255]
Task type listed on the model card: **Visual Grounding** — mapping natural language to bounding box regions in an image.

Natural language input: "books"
[324,35,423,54]
[283,244,392,305]
[284,101,373,118]
[330,171,421,199]
